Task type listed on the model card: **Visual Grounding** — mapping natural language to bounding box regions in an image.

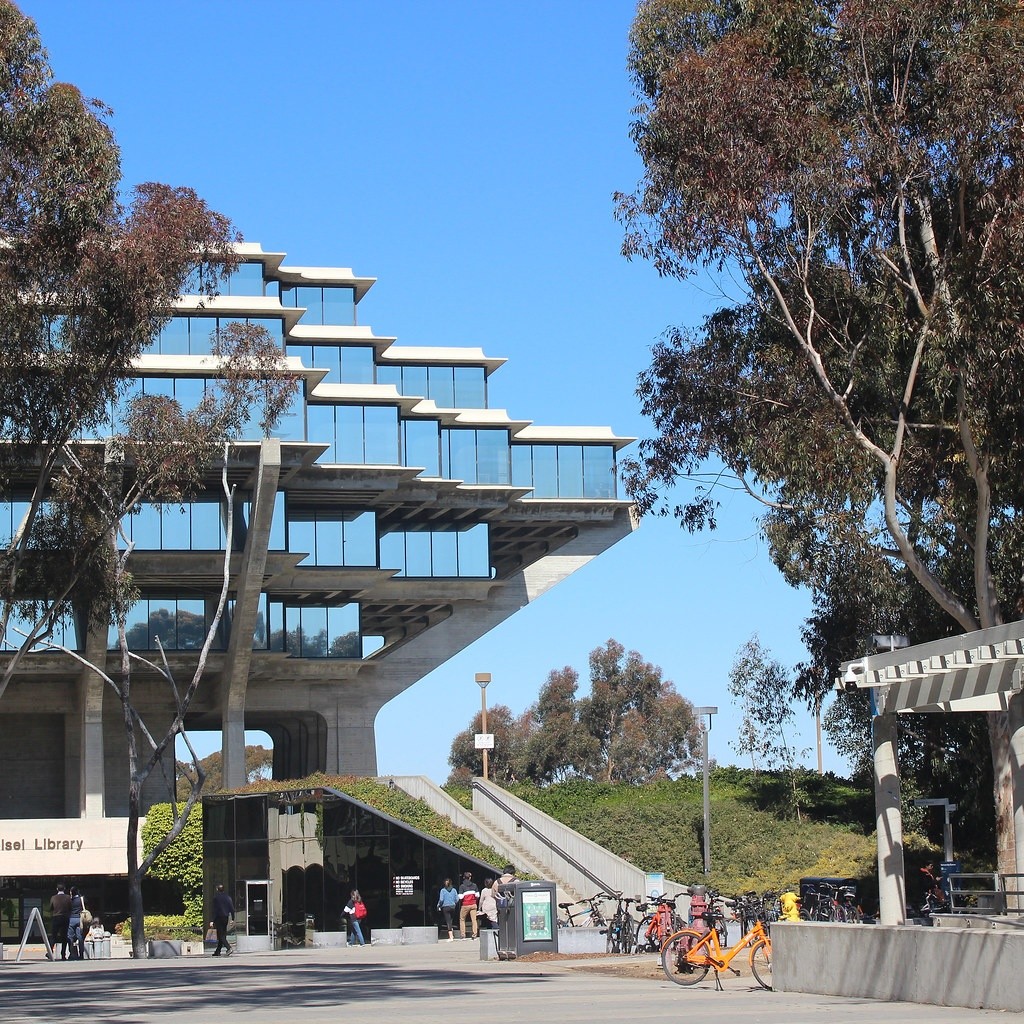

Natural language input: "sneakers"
[212,948,232,957]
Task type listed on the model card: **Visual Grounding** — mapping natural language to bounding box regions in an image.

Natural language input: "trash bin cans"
[493,881,558,961]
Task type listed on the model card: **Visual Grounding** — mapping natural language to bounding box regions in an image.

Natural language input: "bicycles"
[560,880,864,995]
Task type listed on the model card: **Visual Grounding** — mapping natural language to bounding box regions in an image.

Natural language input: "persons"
[430,884,444,936]
[457,872,480,940]
[437,877,459,940]
[915,860,942,917]
[45,883,72,960]
[4,900,17,926]
[491,864,521,925]
[67,885,91,961]
[209,884,236,957]
[340,888,366,947]
[478,878,499,931]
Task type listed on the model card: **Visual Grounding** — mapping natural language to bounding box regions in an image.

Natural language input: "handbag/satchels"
[205,925,217,943]
[81,897,93,925]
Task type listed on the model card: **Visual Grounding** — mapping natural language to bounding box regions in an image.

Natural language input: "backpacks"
[354,901,367,919]
